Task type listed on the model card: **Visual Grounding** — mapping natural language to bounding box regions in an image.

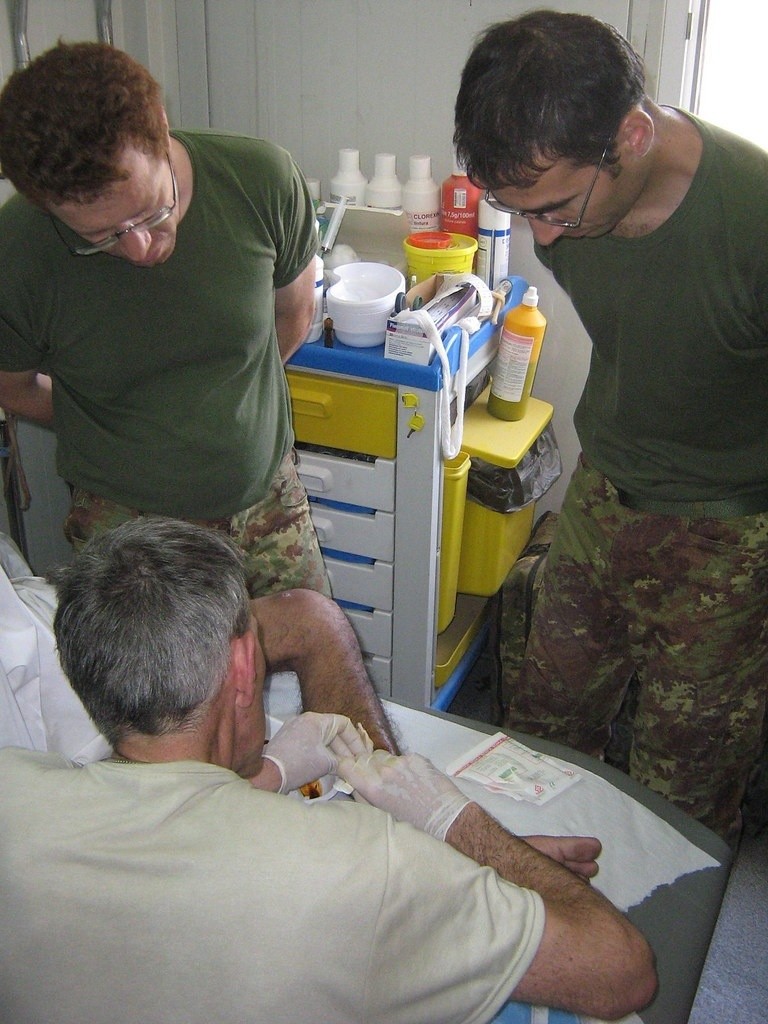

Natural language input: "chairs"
[378,696,733,1024]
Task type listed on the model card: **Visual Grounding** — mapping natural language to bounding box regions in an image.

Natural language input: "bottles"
[439,153,484,244]
[402,155,440,232]
[329,149,368,208]
[477,198,511,291]
[365,153,403,214]
[487,286,547,421]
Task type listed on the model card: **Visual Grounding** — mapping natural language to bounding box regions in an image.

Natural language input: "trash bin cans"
[448,380,554,598]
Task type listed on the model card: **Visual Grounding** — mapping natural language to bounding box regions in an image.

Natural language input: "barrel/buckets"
[402,232,479,291]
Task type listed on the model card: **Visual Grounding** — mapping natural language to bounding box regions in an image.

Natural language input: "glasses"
[49,150,177,257]
[484,122,618,228]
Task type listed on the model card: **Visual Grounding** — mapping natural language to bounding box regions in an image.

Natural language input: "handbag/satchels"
[498,510,560,720]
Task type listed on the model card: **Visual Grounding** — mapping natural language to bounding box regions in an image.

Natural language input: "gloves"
[339,749,476,843]
[261,711,367,796]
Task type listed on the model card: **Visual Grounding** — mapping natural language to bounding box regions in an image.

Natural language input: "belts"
[603,476,768,519]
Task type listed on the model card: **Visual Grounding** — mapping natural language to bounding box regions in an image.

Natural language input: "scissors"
[396,292,424,313]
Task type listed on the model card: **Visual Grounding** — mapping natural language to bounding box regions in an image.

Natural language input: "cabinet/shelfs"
[284,277,529,714]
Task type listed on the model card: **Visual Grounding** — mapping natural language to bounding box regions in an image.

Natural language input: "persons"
[0,42,337,613]
[455,9,765,870]
[0,512,658,1024]
